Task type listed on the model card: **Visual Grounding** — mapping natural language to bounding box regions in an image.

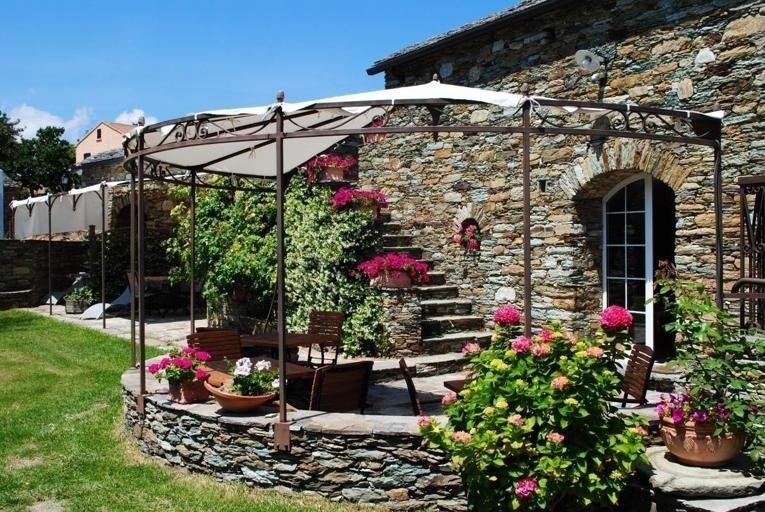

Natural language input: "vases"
[659,417,745,469]
[167,379,207,405]
[204,382,281,414]
[323,165,346,183]
[378,271,411,288]
[369,207,379,222]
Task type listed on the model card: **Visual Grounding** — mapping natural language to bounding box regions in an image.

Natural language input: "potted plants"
[64,286,93,314]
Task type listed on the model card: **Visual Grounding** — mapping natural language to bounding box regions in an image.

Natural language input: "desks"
[243,335,328,364]
[145,275,184,313]
[213,352,315,386]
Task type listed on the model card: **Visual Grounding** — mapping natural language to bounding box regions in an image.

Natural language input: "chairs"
[174,277,205,315]
[306,310,343,366]
[187,328,241,362]
[603,344,653,409]
[124,269,162,317]
[399,357,424,416]
[289,360,375,414]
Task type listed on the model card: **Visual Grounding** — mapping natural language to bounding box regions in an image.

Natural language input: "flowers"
[152,341,212,383]
[300,150,359,188]
[220,354,289,395]
[657,381,758,442]
[349,252,430,283]
[452,225,480,253]
[329,191,389,211]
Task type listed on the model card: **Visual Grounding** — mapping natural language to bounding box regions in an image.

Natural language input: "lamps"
[575,47,602,73]
